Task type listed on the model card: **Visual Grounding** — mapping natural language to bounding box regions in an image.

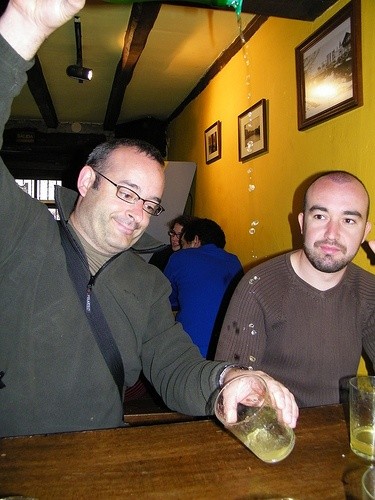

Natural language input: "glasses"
[93,167,165,216]
[167,230,181,238]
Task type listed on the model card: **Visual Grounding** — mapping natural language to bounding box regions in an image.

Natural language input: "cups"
[361,466,375,500]
[349,376,375,461]
[214,374,296,463]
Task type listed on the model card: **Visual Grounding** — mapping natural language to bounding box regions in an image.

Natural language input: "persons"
[214,170,375,407]
[149,216,245,360]
[0,0,299,440]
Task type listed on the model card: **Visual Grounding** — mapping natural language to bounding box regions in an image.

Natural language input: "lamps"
[66,65,93,81]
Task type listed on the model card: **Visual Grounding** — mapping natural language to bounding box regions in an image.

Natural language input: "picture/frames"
[204,121,221,165]
[295,0,364,132]
[238,99,268,162]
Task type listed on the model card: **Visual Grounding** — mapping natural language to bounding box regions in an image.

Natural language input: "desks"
[0,404,375,500]
